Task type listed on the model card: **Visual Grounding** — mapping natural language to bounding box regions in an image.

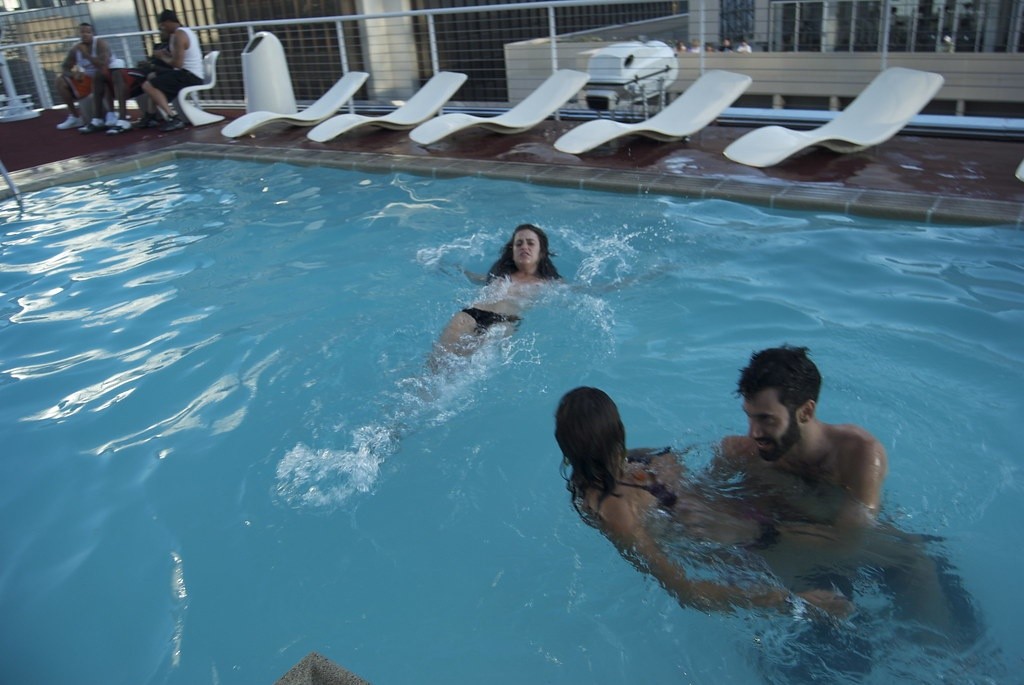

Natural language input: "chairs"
[409,70,591,145]
[79,59,125,122]
[174,51,226,129]
[220,71,370,138]
[306,71,468,144]
[552,70,752,154]
[724,66,945,167]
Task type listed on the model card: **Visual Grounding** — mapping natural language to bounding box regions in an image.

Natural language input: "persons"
[448,222,556,338]
[54,11,202,136]
[673,37,752,53]
[555,388,652,529]
[723,345,883,511]
[938,36,954,53]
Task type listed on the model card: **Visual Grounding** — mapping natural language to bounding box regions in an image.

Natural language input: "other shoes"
[169,114,190,125]
[136,112,158,128]
[105,120,131,134]
[104,112,119,126]
[56,114,84,129]
[159,119,184,132]
[78,117,109,133]
[148,111,166,127]
